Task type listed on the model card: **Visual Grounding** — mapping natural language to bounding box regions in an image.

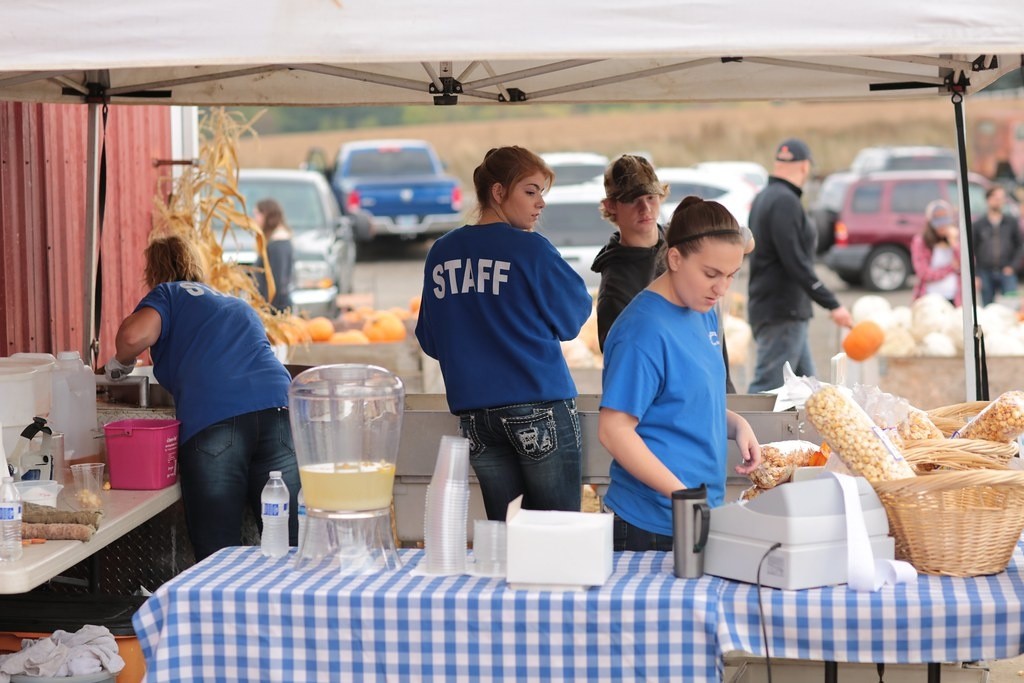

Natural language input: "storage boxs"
[0,587,147,683]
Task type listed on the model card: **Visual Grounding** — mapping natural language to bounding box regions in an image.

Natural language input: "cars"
[303,140,462,245]
[199,167,355,321]
[849,145,957,170]
[522,154,767,291]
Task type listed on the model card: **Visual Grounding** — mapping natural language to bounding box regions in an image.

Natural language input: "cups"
[424,434,507,578]
[70,462,105,511]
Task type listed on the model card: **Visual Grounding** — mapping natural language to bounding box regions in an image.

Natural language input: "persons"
[253,199,294,313]
[598,196,763,553]
[747,140,852,398]
[589,155,756,397]
[910,185,1024,309]
[415,146,592,522]
[105,235,301,565]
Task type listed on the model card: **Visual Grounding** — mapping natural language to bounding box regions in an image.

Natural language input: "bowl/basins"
[13,480,59,509]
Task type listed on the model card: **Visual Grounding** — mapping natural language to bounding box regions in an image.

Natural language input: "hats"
[777,138,814,164]
[603,154,664,203]
[926,200,954,228]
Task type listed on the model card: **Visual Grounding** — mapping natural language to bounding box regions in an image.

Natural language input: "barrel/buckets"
[93,417,181,491]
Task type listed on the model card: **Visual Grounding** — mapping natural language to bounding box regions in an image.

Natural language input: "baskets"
[877,469,1024,577]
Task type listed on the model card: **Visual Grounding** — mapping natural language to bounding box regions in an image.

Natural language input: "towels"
[0,623,128,683]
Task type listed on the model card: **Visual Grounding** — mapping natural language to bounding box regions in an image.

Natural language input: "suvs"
[806,169,1024,294]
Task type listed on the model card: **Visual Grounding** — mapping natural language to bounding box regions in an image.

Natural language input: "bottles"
[260,471,290,559]
[10,344,100,481]
[0,476,24,563]
[298,487,308,554]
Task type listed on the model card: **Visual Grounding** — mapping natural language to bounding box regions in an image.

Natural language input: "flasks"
[671,483,711,579]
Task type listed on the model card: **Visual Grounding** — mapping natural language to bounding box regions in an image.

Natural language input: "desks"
[0,479,180,594]
[132,548,1024,683]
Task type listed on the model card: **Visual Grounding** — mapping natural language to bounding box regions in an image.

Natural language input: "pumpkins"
[842,294,1024,361]
[290,296,421,345]
